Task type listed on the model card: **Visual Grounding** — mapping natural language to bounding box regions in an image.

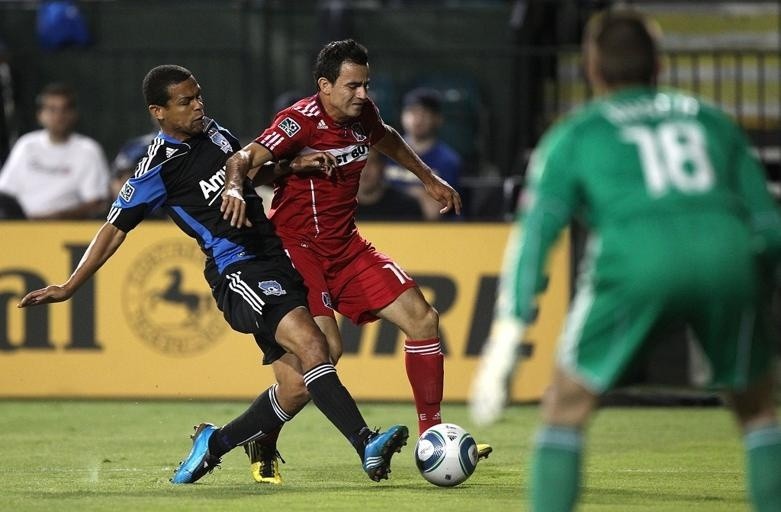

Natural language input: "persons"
[13,64,412,487]
[216,38,494,487]
[109,127,161,216]
[378,85,466,224]
[0,78,109,222]
[347,149,424,222]
[467,9,781,510]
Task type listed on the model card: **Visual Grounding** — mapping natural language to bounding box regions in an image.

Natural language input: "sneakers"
[243,438,285,485]
[168,422,222,483]
[359,424,409,482]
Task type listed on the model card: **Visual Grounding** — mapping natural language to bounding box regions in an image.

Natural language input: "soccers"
[415,423,478,487]
